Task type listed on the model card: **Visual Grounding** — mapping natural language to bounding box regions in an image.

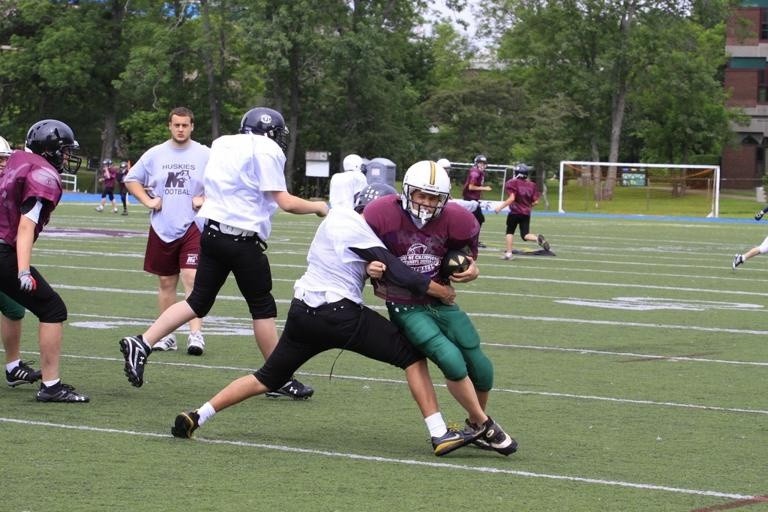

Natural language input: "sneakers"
[7,361,44,388]
[732,253,743,272]
[36,381,89,402]
[119,333,151,388]
[171,411,200,440]
[122,211,127,215]
[186,331,204,355]
[109,208,117,213]
[501,254,512,260]
[153,334,178,351]
[479,242,485,247]
[96,206,103,211]
[537,233,551,252]
[264,375,314,400]
[754,213,763,220]
[467,416,518,456]
[431,427,488,457]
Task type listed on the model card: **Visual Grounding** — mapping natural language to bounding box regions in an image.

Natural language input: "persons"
[94,157,119,216]
[328,152,369,213]
[732,203,768,269]
[171,182,486,457]
[362,159,520,457]
[118,107,328,402]
[494,164,551,262]
[0,119,92,404]
[116,161,131,217]
[459,154,492,250]
[123,108,210,356]
[0,135,12,177]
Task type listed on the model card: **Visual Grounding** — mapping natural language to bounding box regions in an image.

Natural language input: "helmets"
[437,158,451,169]
[343,154,366,172]
[404,161,452,221]
[119,162,127,172]
[355,183,399,214]
[103,159,113,168]
[474,155,488,171]
[0,136,13,156]
[25,120,81,175]
[240,107,289,151]
[515,163,525,180]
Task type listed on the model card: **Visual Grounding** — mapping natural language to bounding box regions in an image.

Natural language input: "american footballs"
[441,249,470,280]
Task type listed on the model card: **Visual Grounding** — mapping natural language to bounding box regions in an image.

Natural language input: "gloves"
[18,270,37,291]
